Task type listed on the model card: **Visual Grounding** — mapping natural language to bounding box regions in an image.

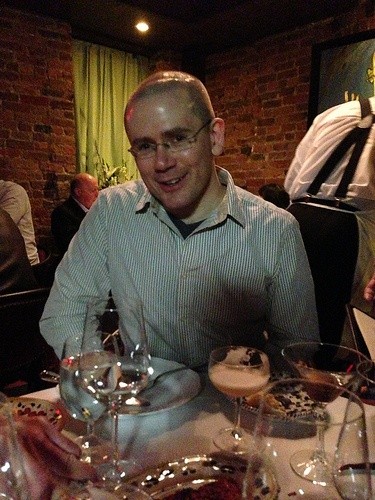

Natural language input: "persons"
[40,71,323,374]
[284,96,375,362]
[0,181,41,294]
[257,183,310,246]
[51,174,100,253]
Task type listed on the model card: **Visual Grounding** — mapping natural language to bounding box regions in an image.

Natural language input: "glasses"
[127,119,212,159]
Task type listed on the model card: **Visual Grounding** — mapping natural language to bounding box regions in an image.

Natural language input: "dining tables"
[0,386,375,500]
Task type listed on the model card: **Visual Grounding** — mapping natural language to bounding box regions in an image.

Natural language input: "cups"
[0,393,30,500]
[241,379,372,500]
[332,361,375,500]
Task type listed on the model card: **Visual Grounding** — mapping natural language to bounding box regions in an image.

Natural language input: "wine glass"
[207,346,270,455]
[58,331,122,463]
[80,296,151,483]
[281,340,373,486]
[88,398,170,500]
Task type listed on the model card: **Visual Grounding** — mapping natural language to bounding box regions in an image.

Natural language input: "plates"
[117,454,279,500]
[149,355,200,415]
[232,399,330,439]
[8,397,65,433]
